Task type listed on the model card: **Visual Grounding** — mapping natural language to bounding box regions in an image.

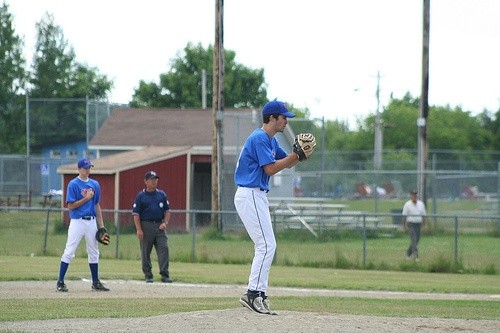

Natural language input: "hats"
[143,171,159,180]
[410,190,418,195]
[77,158,95,169]
[261,98,296,118]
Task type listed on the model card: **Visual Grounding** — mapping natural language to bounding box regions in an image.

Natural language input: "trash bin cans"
[390,208,404,224]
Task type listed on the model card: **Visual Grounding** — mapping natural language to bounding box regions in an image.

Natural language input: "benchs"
[269,196,398,240]
[0,189,62,212]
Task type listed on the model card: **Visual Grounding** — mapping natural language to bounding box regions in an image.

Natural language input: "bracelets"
[163,220,169,224]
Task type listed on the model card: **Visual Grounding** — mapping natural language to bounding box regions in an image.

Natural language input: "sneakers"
[91,279,110,292]
[56,280,69,292]
[262,295,278,316]
[239,290,272,315]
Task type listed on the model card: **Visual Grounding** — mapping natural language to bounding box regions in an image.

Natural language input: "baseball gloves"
[95,227,110,245]
[292,133,316,162]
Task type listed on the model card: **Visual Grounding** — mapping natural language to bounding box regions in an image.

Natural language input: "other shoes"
[161,277,173,283]
[404,252,411,260]
[414,257,420,262]
[146,278,154,283]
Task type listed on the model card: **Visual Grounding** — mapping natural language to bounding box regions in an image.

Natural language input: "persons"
[233,101,317,316]
[56,157,111,291]
[402,191,426,262]
[132,171,172,283]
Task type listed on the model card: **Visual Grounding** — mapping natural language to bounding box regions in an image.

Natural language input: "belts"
[239,185,269,193]
[82,217,95,220]
